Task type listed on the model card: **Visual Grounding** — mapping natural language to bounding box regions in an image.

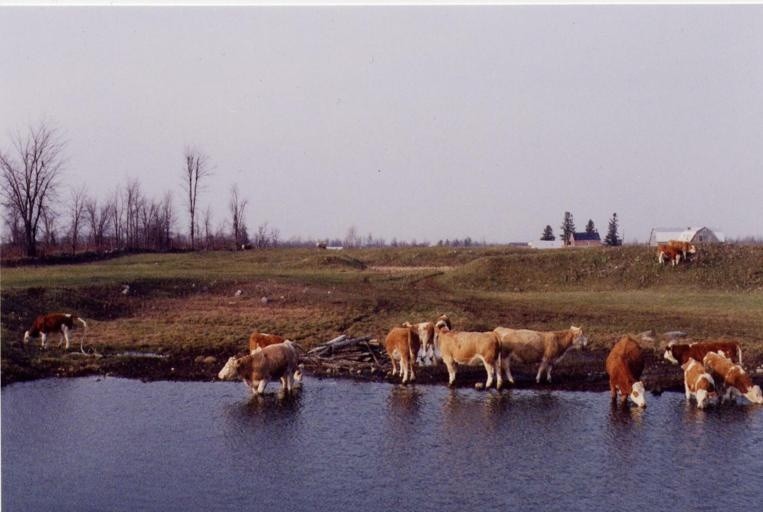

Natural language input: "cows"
[248,331,305,382]
[383,314,455,384]
[21,312,85,351]
[656,239,697,268]
[664,338,763,410]
[433,323,504,392]
[605,334,648,410]
[217,340,300,403]
[493,324,591,383]
